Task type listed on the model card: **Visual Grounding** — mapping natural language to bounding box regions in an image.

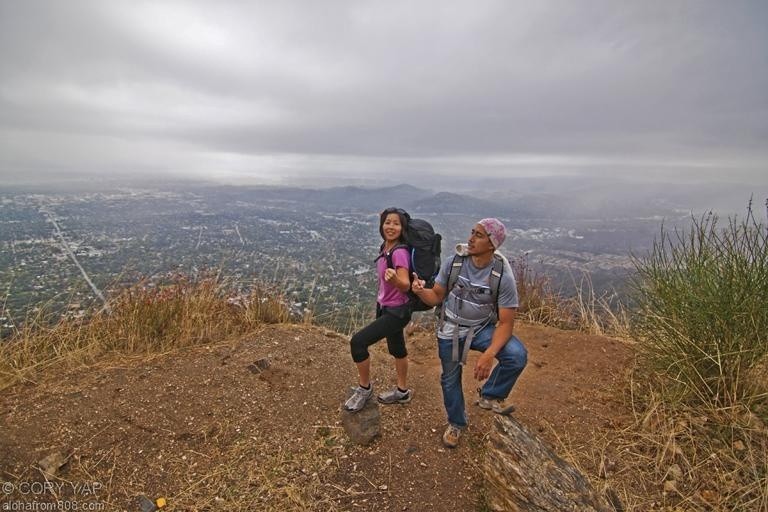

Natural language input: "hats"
[477,218,507,250]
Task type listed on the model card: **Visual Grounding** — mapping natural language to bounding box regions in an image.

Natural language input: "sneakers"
[480,397,516,414]
[377,387,412,404]
[344,382,374,412]
[442,424,463,447]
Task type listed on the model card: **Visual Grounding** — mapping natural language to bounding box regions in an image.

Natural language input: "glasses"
[386,207,406,215]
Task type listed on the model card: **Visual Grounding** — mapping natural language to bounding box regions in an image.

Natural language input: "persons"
[409,217,529,449]
[342,207,414,415]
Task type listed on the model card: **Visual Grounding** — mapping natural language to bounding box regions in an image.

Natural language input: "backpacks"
[374,218,442,312]
